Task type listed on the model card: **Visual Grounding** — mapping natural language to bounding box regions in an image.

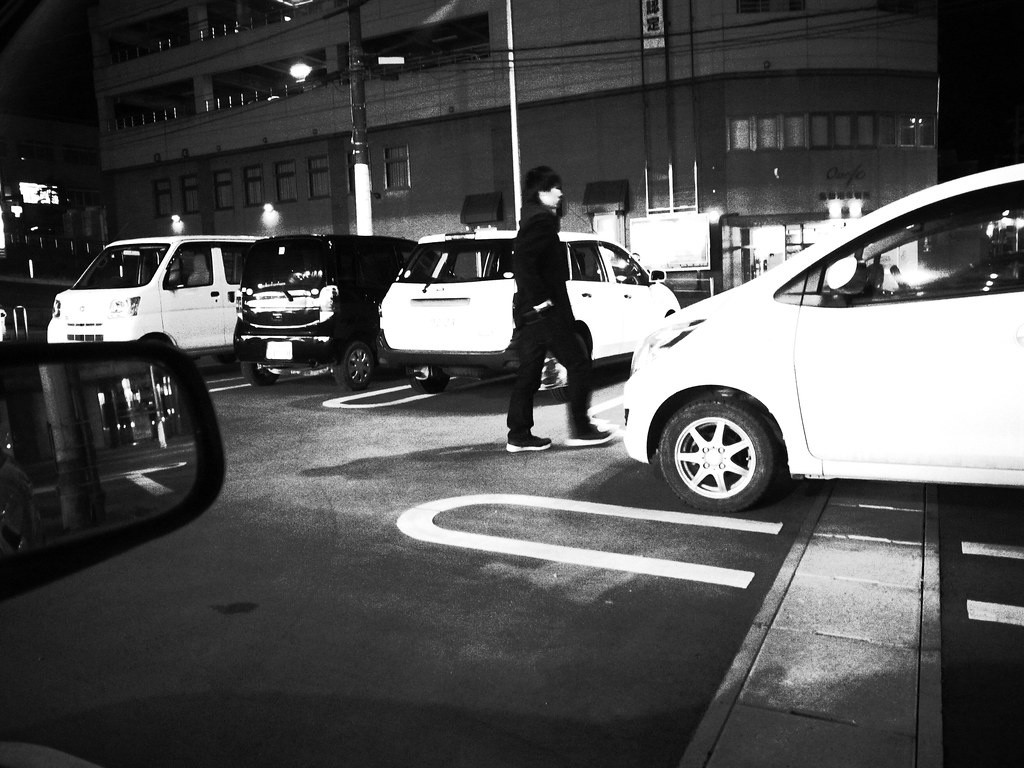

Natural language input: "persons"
[506,166,612,450]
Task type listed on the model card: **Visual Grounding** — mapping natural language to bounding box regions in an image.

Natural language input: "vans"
[237,229,461,392]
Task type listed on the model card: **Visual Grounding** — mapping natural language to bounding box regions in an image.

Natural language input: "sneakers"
[567,424,615,447]
[507,434,552,452]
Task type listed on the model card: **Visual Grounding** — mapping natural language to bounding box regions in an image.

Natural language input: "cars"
[46,235,280,367]
[625,165,1024,496]
[377,226,681,394]
